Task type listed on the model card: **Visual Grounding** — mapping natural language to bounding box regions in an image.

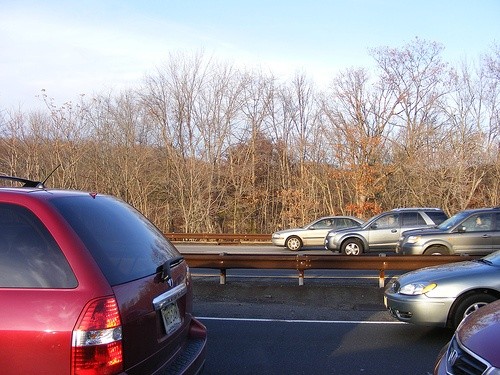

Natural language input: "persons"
[474,215,490,230]
[326,219,333,227]
[387,216,397,226]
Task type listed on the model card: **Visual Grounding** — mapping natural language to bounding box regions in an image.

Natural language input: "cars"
[382,249,500,332]
[432,299,500,375]
[271,216,367,251]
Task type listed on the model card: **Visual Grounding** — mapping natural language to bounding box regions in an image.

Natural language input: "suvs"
[395,207,500,256]
[323,207,448,256]
[0,176,209,375]
